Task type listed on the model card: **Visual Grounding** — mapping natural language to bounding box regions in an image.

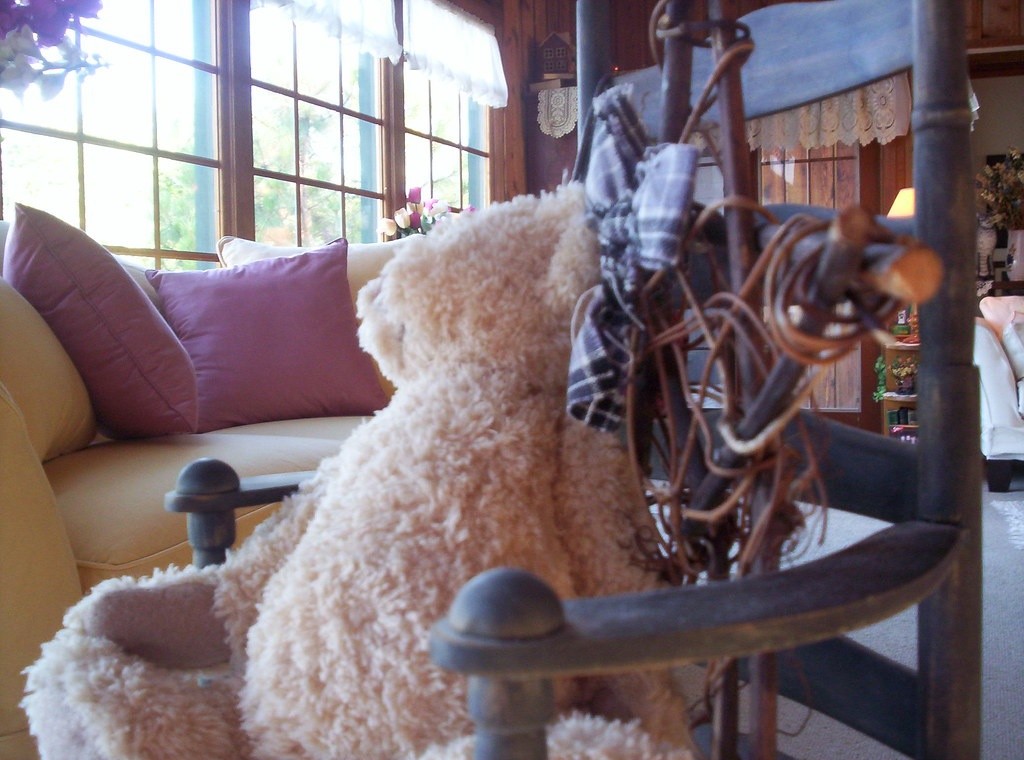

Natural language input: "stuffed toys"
[28,186,696,760]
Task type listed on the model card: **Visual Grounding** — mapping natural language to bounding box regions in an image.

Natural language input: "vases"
[975,228,997,279]
[1005,229,1024,281]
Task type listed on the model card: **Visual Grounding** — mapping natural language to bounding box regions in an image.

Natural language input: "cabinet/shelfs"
[881,340,921,438]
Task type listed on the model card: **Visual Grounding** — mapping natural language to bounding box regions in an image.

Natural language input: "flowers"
[976,145,1024,231]
[377,188,475,238]
[0,0,114,109]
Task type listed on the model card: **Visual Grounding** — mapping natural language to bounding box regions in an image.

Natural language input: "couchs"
[972,319,1024,492]
[0,280,372,760]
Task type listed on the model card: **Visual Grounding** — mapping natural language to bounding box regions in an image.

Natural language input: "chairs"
[20,0,980,760]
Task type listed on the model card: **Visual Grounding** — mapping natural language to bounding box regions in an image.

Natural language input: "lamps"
[886,188,921,343]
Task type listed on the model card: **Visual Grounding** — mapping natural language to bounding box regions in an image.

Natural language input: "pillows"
[3,202,199,440]
[144,241,389,433]
[1001,311,1024,380]
[216,234,427,397]
